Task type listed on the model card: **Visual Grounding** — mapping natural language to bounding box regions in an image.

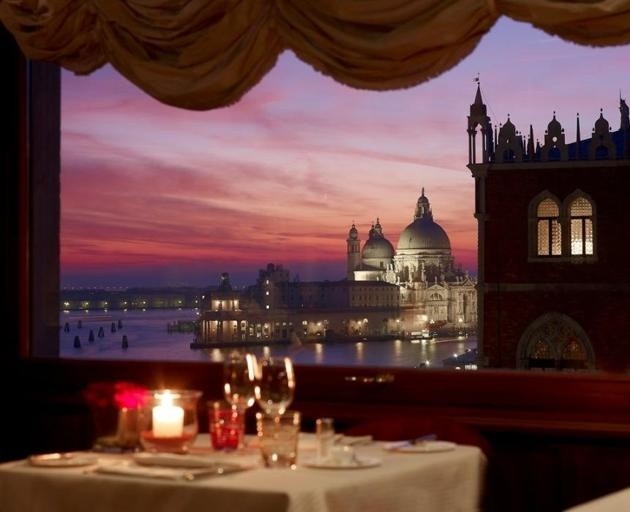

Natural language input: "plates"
[27,454,97,468]
[385,440,457,456]
[93,457,183,478]
[308,459,384,471]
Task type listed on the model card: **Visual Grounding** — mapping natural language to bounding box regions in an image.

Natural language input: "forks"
[390,433,438,448]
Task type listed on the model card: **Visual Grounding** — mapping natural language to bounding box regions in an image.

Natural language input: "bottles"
[316,416,336,460]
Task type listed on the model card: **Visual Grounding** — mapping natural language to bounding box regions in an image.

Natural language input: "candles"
[151,391,186,437]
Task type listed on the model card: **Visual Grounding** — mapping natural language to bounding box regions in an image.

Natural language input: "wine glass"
[221,351,261,452]
[252,354,297,424]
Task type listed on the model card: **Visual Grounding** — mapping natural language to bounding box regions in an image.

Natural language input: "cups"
[132,388,204,455]
[253,410,302,469]
[206,398,247,451]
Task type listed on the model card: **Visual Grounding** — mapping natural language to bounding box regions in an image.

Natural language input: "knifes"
[183,465,254,478]
